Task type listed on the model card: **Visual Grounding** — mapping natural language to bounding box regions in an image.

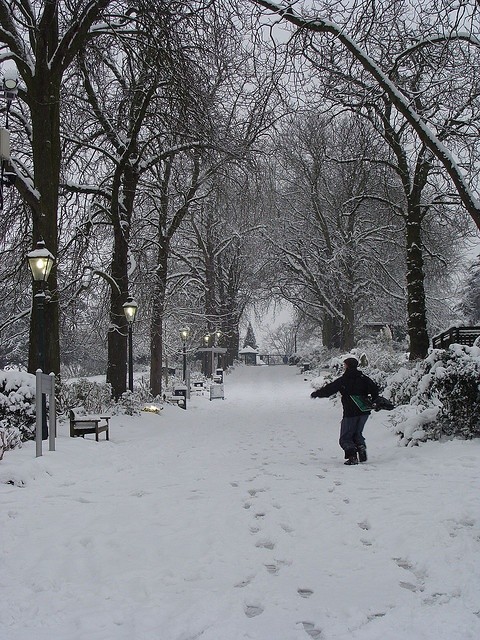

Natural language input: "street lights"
[120,296,140,396]
[23,234,56,441]
[179,326,190,384]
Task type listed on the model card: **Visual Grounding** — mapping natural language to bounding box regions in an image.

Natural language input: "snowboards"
[349,394,394,412]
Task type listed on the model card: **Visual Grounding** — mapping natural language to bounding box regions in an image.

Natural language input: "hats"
[343,358,358,368]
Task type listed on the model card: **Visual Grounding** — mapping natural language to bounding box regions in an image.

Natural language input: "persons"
[310,357,380,465]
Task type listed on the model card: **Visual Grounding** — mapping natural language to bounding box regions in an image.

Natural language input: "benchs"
[70,405,110,442]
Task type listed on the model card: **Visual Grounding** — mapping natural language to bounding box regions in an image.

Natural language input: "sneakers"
[358,445,367,462]
[344,456,359,465]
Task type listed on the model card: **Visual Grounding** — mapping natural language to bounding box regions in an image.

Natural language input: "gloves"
[311,392,318,398]
[374,404,382,412]
